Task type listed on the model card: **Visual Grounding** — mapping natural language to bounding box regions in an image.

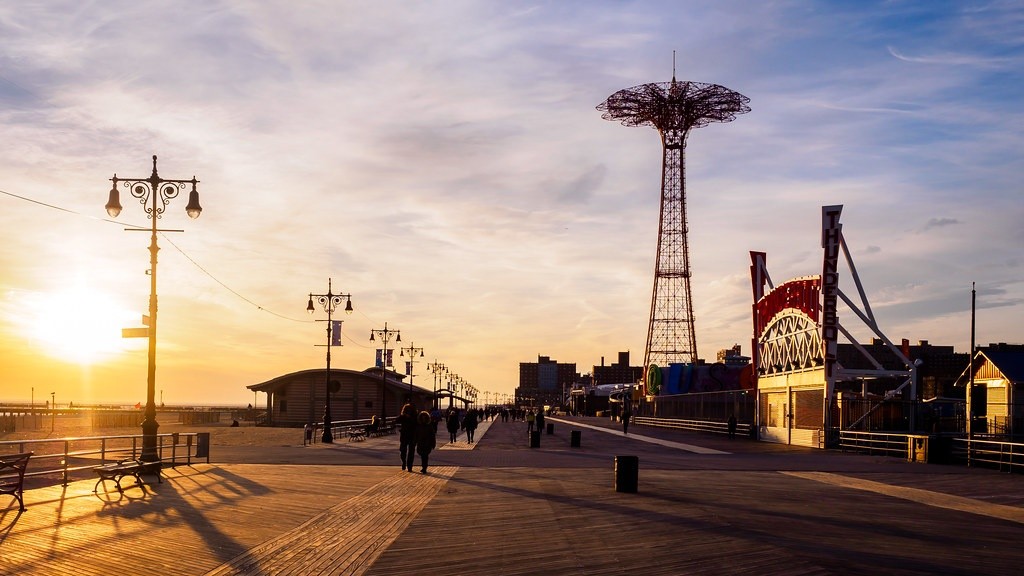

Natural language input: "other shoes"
[421,468,426,473]
[401,463,406,470]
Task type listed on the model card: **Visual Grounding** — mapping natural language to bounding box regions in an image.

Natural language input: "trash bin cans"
[570,430,581,446]
[547,423,553,433]
[197,433,209,457]
[615,456,638,492]
[529,431,540,448]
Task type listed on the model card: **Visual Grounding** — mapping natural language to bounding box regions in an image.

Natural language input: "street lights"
[400,342,425,403]
[484,391,490,405]
[307,277,353,443]
[493,392,500,405]
[502,393,508,404]
[369,322,401,431]
[105,154,202,476]
[426,357,480,411]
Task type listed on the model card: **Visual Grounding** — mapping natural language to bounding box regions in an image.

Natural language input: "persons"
[445,407,501,422]
[548,408,551,417]
[536,411,544,434]
[501,408,544,423]
[446,411,460,443]
[612,406,618,421]
[526,410,535,433]
[727,413,737,439]
[581,408,585,417]
[415,411,436,474]
[394,403,417,472]
[463,409,477,444]
[622,410,631,433]
[416,408,441,432]
[230,419,239,427]
[365,415,380,438]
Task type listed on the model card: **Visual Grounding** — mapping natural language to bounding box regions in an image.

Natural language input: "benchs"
[348,431,366,442]
[92,461,163,493]
[0,450,34,511]
[369,426,396,438]
